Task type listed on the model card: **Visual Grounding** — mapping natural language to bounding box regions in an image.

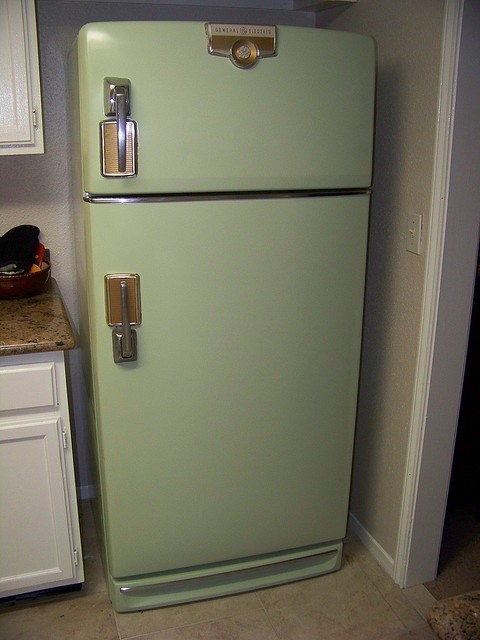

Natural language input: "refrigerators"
[68,20,377,613]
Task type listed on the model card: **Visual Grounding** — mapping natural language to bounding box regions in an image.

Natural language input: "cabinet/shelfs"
[0,351,84,600]
[0,1,46,156]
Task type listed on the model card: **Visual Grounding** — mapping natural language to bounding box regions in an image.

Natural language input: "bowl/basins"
[0,260,51,300]
